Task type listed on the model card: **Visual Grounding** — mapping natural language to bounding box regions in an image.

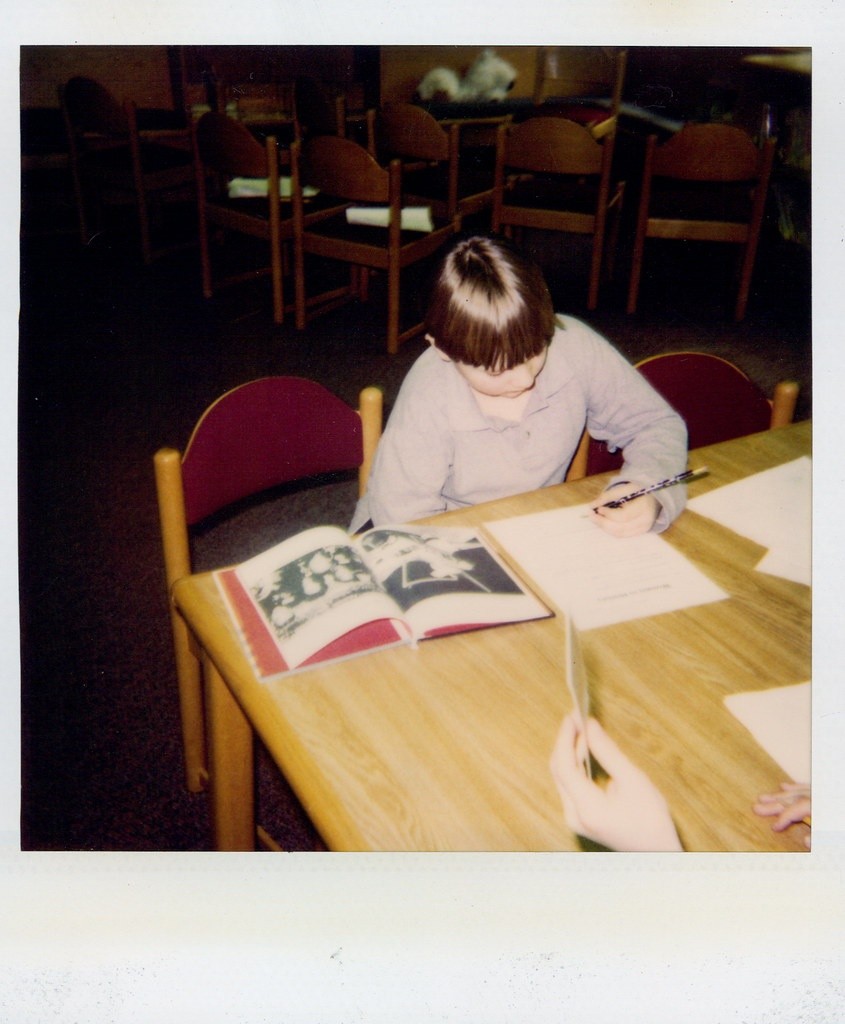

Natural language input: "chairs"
[154,375,383,791]
[380,100,514,240]
[124,98,245,265]
[193,110,360,324]
[563,351,799,483]
[626,123,778,320]
[492,118,626,309]
[59,76,167,249]
[21,106,87,248]
[290,136,456,355]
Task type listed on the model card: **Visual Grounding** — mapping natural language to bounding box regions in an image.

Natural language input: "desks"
[174,418,811,853]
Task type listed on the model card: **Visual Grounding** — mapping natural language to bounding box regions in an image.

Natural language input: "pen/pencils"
[770,792,810,828]
[583,465,709,518]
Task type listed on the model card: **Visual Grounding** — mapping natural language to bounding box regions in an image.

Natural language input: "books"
[213,525,555,683]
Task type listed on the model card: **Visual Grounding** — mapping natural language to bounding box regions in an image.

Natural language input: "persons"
[366,230,688,538]
[552,713,812,853]
[250,546,373,629]
